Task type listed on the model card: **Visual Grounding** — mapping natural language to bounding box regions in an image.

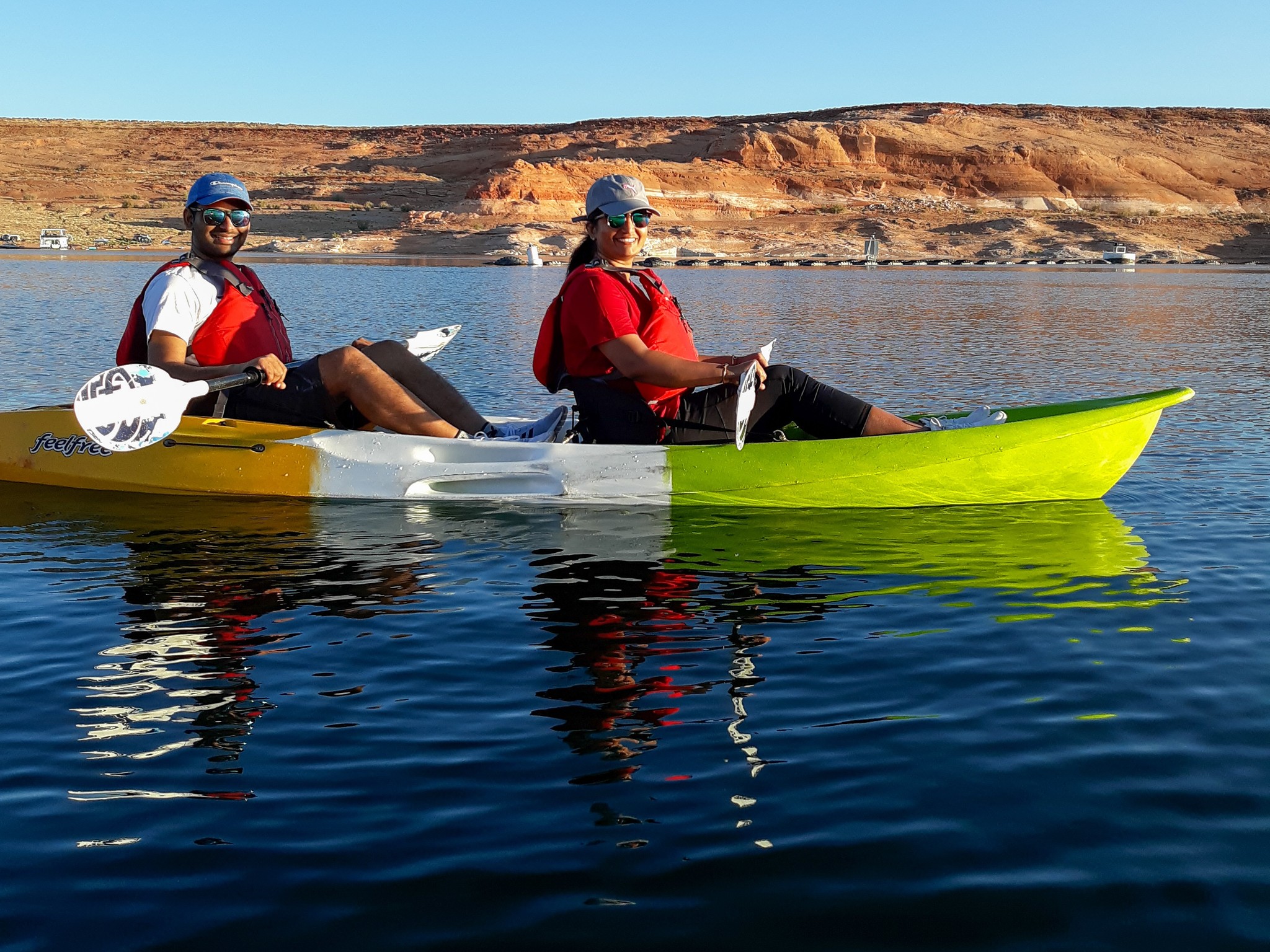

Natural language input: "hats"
[186,173,254,211]
[571,174,661,223]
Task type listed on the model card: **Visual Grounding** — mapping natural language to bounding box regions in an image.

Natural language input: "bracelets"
[720,364,729,384]
[732,355,737,365]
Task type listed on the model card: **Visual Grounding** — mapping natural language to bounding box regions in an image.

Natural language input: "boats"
[0,386,1195,510]
[39,229,74,250]
[1103,243,1137,265]
[0,239,20,249]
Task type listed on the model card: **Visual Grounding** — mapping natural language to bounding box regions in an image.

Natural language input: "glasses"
[190,207,251,227]
[593,212,649,229]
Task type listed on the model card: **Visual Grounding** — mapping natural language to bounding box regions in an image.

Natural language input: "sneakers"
[929,410,1007,432]
[918,405,991,426]
[474,432,556,443]
[497,406,568,438]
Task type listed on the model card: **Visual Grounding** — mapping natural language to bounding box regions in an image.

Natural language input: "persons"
[532,173,1007,445]
[115,173,568,443]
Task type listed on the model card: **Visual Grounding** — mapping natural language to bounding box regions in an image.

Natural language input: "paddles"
[73,324,462,453]
[735,337,777,451]
